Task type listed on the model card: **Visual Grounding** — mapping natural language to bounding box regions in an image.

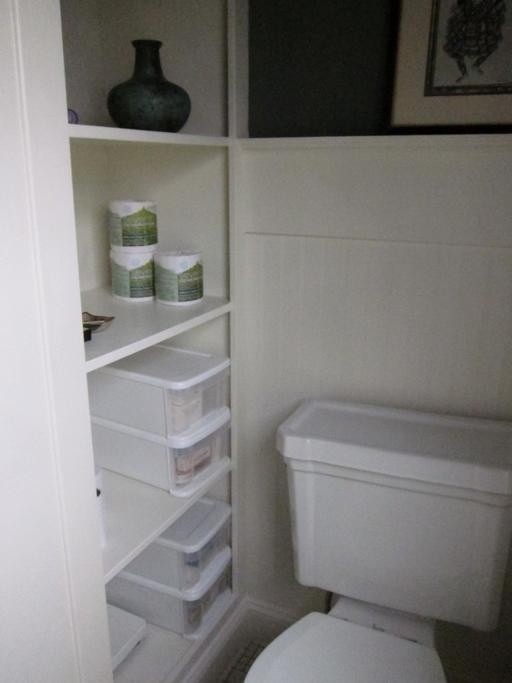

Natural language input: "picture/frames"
[385,0,512,133]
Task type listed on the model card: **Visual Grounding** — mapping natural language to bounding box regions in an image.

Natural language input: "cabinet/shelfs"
[59,0,247,683]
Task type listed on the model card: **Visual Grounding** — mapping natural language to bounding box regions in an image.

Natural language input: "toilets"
[243,401,512,682]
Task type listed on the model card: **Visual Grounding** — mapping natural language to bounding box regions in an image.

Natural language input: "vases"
[108,39,191,132]
